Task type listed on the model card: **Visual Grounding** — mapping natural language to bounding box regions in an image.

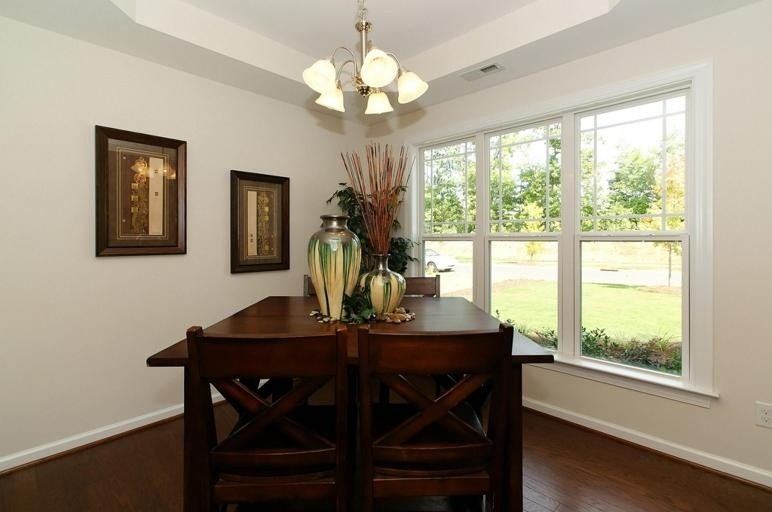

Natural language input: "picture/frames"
[95,125,187,256]
[230,170,290,273]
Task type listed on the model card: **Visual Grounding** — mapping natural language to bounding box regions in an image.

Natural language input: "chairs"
[404,275,440,298]
[184,327,348,512]
[357,324,513,511]
[308,215,362,323]
[304,275,316,295]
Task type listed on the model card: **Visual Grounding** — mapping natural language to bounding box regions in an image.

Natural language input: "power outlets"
[754,402,771,428]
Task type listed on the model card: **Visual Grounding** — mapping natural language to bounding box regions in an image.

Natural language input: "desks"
[146,296,554,511]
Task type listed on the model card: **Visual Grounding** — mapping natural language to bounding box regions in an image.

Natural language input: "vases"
[357,254,406,321]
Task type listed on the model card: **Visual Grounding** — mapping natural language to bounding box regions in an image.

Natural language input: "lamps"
[301,1,429,115]
